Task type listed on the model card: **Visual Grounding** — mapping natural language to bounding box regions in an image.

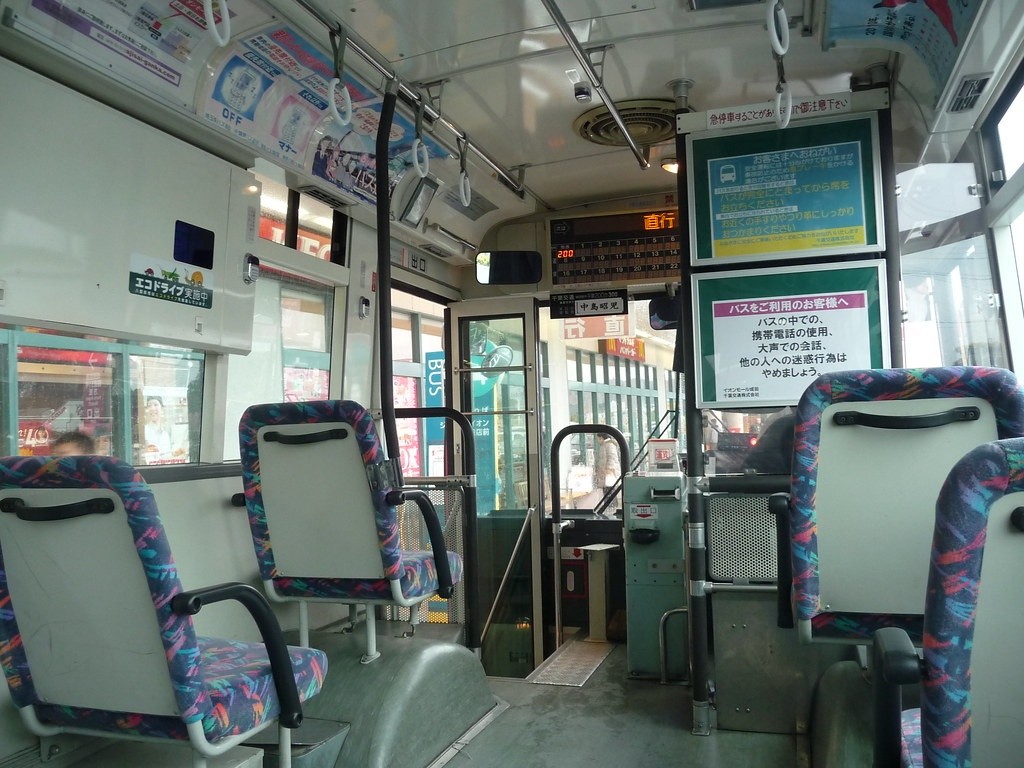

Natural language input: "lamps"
[660,159,678,174]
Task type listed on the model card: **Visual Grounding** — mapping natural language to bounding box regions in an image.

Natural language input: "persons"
[594,431,626,508]
[52,431,98,460]
[145,396,172,458]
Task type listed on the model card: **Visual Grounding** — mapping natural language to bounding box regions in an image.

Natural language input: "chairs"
[0,458,328,766]
[237,398,466,663]
[788,356,1023,767]
